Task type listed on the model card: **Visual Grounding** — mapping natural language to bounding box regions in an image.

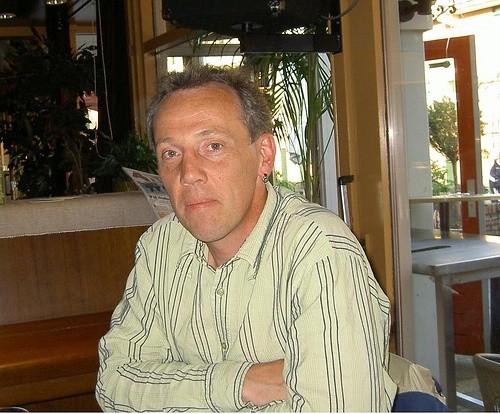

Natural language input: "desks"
[411,228,500,412]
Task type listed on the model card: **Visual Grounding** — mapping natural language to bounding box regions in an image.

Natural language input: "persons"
[95,59,397,413]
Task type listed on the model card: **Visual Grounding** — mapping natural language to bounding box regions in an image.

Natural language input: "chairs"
[472,351,500,413]
